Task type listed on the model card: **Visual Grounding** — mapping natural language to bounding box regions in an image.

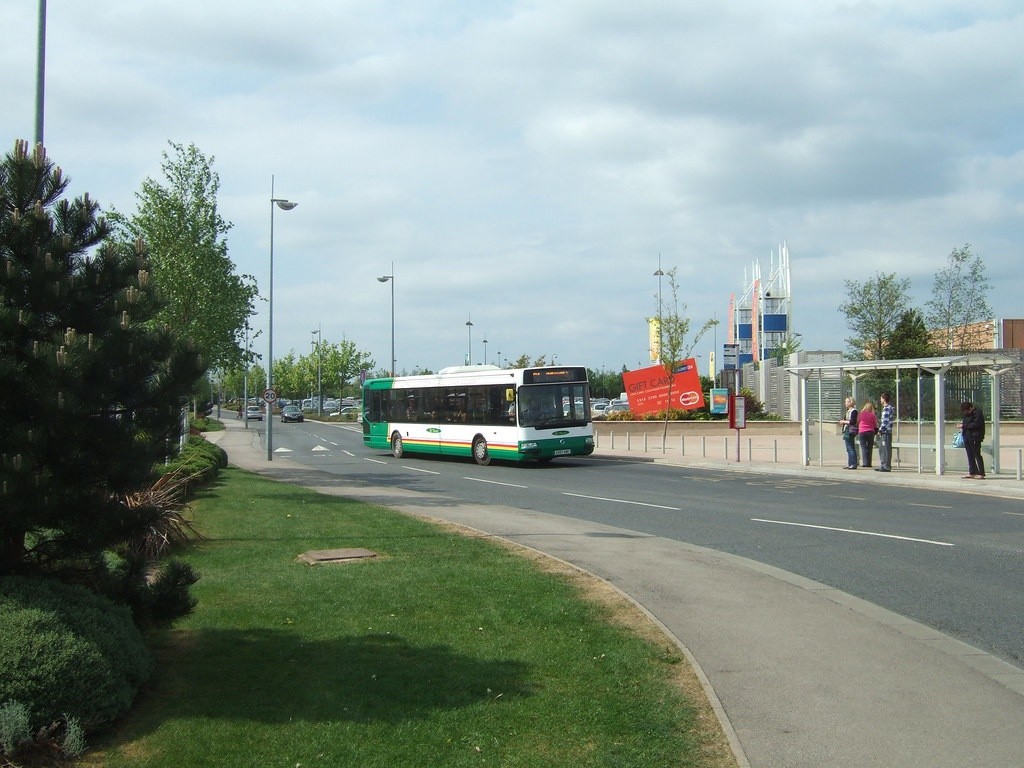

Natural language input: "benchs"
[855,441,994,458]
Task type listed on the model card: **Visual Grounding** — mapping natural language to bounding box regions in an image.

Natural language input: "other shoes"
[875,467,890,472]
[844,465,849,469]
[975,474,983,478]
[961,474,973,478]
[849,465,857,469]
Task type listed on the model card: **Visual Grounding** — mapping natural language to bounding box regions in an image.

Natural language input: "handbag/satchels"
[849,424,858,437]
[843,428,852,441]
[952,430,966,448]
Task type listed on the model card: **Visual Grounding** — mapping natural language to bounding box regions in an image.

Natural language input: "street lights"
[481,333,508,365]
[265,175,298,454]
[653,252,665,366]
[465,310,474,364]
[376,259,396,379]
[311,321,324,418]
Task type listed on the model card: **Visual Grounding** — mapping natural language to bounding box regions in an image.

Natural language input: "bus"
[361,365,595,467]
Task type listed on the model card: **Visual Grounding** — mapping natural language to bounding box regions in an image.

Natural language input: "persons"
[872,392,894,472]
[839,396,859,470]
[956,402,985,479]
[857,403,879,467]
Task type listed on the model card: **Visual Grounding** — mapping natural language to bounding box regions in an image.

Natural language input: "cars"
[225,396,360,417]
[560,396,630,420]
[280,405,304,423]
[246,405,263,420]
